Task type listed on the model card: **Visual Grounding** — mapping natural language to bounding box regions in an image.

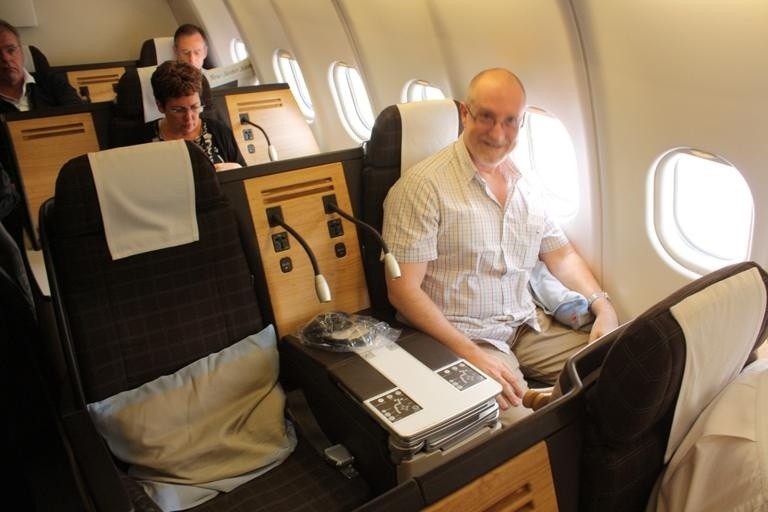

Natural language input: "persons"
[647,359,768,512]
[169,23,210,71]
[140,60,247,172]
[380,67,620,425]
[0,20,91,188]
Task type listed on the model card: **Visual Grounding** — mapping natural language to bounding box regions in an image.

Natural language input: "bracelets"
[587,291,612,311]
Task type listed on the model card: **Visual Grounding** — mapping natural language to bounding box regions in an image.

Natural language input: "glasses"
[467,105,525,128]
[168,102,206,112]
